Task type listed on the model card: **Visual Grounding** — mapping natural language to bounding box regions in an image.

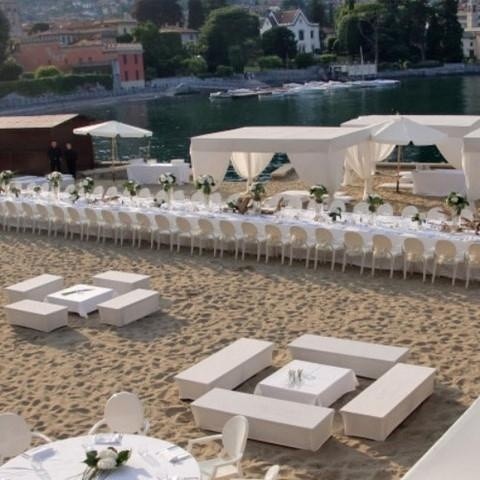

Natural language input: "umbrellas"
[364,117,448,193]
[73,120,153,166]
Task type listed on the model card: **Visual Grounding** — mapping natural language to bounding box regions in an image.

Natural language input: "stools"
[271,163,293,177]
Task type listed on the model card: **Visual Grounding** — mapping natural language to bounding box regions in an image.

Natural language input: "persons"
[47,140,62,172]
[64,142,77,179]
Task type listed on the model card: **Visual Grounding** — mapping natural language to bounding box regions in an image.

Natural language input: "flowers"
[0,169,266,201]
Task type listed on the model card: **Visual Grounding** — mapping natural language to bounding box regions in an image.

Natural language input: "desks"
[413,168,467,196]
[44,284,118,319]
[129,163,190,186]
[254,359,360,407]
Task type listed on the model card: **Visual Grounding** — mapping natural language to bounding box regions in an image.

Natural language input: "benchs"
[96,288,160,327]
[93,270,150,295]
[287,333,410,380]
[340,363,436,442]
[173,336,274,401]
[5,273,64,305]
[4,299,68,332]
[191,388,334,452]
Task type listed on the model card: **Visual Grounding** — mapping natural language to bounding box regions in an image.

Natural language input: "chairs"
[0,392,280,480]
[147,159,157,163]
[171,159,185,163]
